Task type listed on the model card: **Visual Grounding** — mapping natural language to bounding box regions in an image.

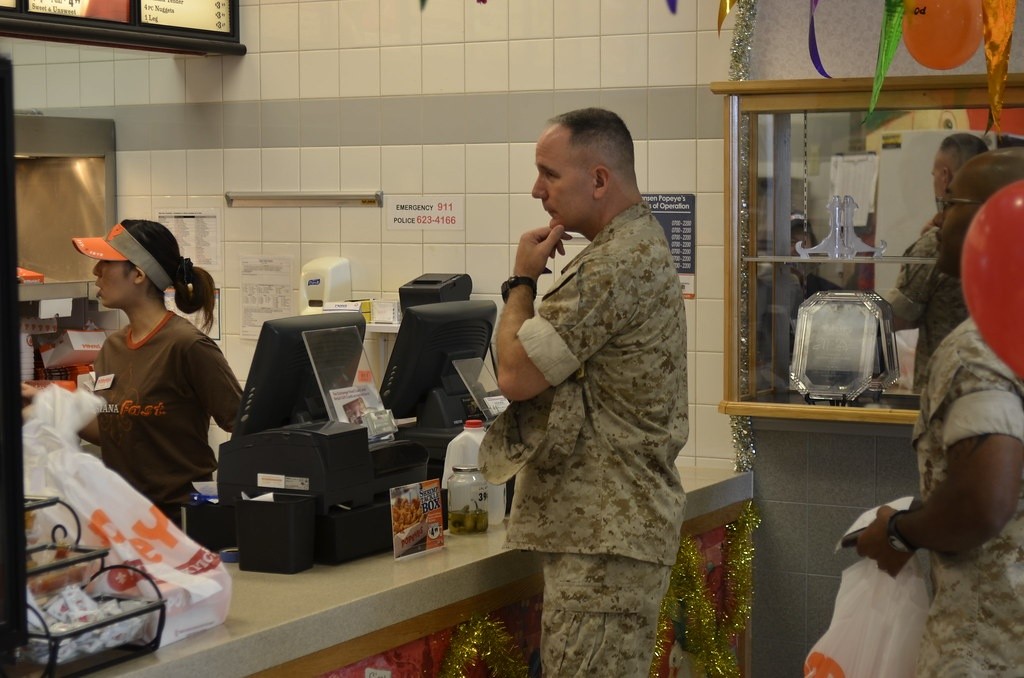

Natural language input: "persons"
[757,134,987,397]
[20,219,244,517]
[856,145,1024,678]
[496,107,688,678]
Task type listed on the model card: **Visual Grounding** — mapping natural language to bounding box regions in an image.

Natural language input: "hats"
[72,223,173,292]
[479,378,585,486]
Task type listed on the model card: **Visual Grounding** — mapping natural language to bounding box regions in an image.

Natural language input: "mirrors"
[717,72,1024,426]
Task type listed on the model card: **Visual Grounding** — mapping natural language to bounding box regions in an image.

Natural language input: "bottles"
[447,466,489,534]
[440,420,507,526]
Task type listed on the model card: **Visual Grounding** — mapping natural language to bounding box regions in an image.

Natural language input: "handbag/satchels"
[22,384,231,647]
[804,553,928,678]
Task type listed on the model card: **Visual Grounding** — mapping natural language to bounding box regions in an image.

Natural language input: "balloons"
[962,180,1024,378]
[901,0,985,70]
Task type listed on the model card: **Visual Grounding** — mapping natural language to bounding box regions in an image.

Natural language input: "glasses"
[936,197,983,214]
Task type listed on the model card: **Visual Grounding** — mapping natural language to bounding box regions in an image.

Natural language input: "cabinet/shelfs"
[0,496,167,678]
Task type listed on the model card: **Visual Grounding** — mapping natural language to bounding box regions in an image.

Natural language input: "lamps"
[225,188,383,208]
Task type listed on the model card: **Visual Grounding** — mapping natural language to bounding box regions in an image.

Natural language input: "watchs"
[500,275,538,303]
[886,511,916,553]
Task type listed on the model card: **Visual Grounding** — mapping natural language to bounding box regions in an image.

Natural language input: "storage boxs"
[232,492,318,574]
[38,319,106,371]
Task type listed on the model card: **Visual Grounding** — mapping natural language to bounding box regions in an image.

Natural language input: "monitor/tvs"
[230,312,366,438]
[379,301,498,416]
